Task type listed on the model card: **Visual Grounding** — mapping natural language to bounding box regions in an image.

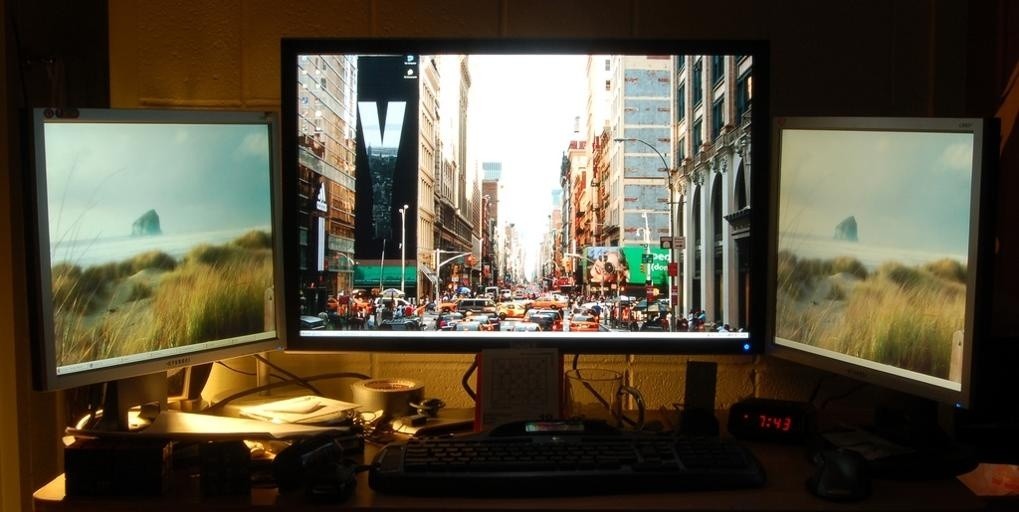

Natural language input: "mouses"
[807,449,872,501]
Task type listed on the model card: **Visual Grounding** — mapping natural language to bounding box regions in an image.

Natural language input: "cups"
[565,368,644,432]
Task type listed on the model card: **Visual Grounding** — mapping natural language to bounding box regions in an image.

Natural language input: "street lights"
[611,136,677,331]
[565,254,605,301]
[633,226,653,302]
[397,203,409,296]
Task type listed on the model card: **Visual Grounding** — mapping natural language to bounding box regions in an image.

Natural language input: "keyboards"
[367,434,766,499]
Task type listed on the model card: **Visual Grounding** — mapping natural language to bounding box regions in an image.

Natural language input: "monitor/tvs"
[281,36,770,431]
[17,106,289,442]
[766,114,1000,484]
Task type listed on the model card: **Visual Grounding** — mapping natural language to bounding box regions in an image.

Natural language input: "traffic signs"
[641,254,655,265]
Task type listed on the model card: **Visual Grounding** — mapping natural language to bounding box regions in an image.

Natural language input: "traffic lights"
[639,263,644,274]
[467,255,474,267]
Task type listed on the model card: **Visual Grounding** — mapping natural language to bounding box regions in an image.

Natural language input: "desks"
[32,409,986,512]
